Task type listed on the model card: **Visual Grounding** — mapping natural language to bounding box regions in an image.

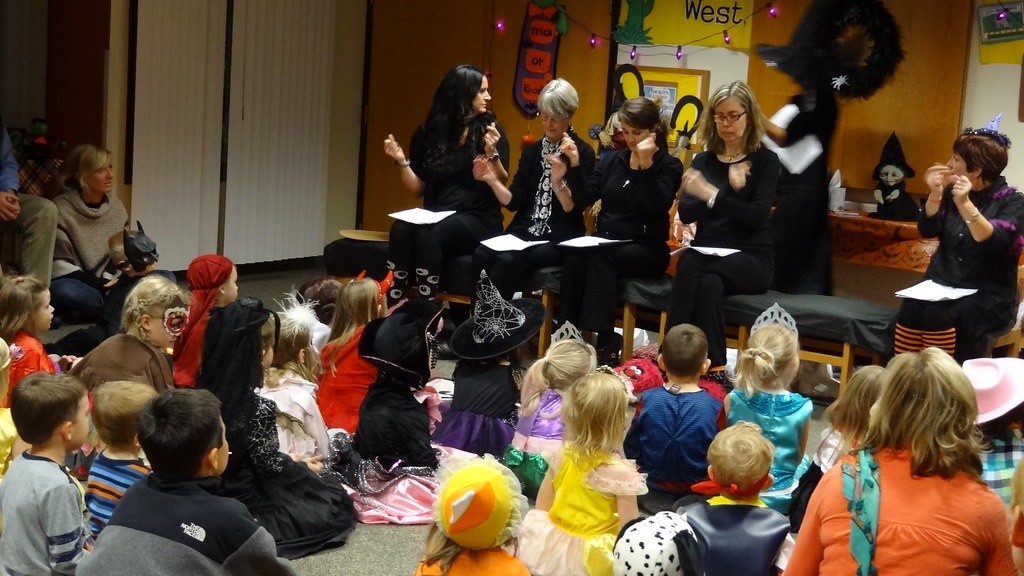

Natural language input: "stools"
[322,238,1024,399]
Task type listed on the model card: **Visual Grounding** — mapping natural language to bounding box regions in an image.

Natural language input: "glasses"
[536,112,555,122]
[713,111,747,122]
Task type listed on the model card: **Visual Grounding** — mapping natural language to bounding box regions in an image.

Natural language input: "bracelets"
[707,188,719,209]
[551,181,567,192]
[394,158,411,168]
[927,194,943,202]
[965,208,980,224]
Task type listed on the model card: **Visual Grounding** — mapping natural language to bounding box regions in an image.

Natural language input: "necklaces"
[725,150,741,162]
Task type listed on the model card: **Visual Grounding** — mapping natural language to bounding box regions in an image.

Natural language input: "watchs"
[488,152,499,162]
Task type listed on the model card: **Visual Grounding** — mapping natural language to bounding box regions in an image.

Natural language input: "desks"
[828,211,938,273]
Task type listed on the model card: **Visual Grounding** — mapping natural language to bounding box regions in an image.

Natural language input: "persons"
[559,97,683,372]
[470,77,597,319]
[768,76,834,295]
[0,124,1023,576]
[658,81,781,369]
[384,65,509,305]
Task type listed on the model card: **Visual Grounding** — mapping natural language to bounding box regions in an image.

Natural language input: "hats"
[961,357,1024,425]
[430,453,529,549]
[358,300,445,388]
[450,269,547,360]
